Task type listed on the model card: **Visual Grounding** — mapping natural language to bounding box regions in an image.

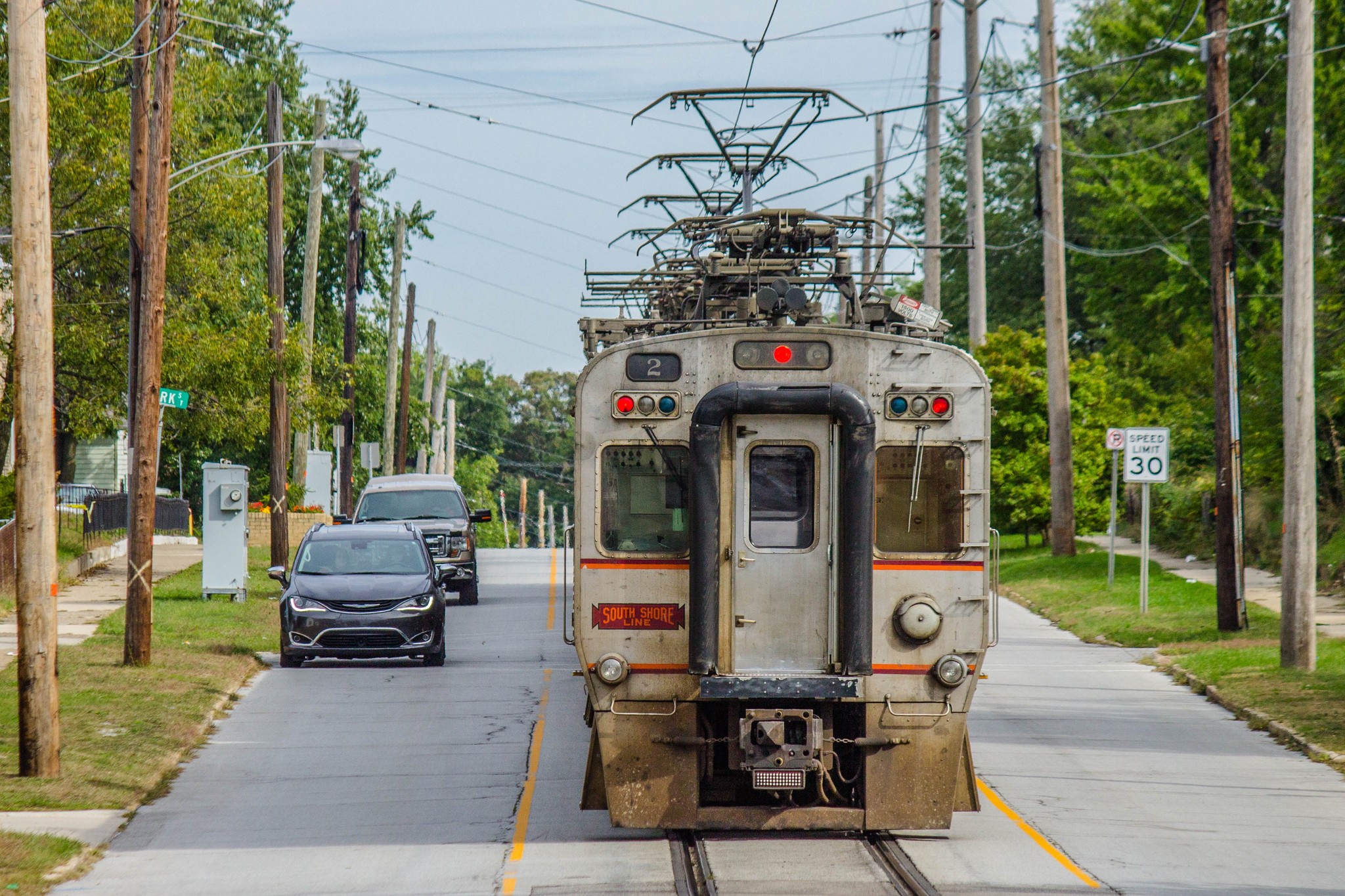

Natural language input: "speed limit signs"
[1124,429,1169,483]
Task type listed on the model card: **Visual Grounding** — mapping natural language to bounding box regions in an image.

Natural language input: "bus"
[563,84,1003,834]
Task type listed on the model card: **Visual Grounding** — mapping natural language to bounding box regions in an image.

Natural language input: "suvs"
[333,473,491,606]
[266,521,459,667]
